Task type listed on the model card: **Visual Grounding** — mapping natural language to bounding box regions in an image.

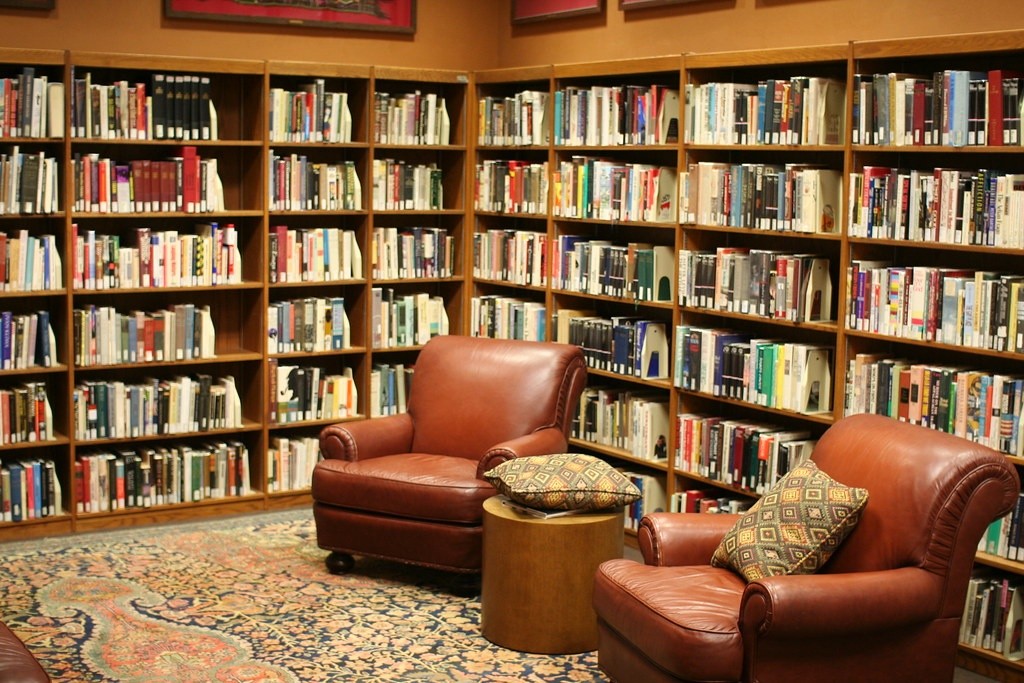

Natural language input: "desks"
[482,494,624,656]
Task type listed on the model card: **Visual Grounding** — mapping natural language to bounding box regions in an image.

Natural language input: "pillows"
[483,453,644,511]
[710,456,868,583]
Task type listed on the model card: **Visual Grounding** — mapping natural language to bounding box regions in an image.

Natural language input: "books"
[0,73,251,524]
[467,71,1023,665]
[267,78,454,497]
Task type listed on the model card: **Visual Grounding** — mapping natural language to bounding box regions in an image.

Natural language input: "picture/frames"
[164,0,417,36]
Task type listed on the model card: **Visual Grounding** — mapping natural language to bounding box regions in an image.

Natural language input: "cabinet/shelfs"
[0,47,475,544]
[509,0,603,26]
[467,29,1024,683]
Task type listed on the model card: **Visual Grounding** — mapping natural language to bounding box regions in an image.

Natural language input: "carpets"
[0,507,610,682]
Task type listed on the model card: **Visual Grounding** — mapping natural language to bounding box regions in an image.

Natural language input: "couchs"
[593,413,1019,683]
[311,336,586,600]
